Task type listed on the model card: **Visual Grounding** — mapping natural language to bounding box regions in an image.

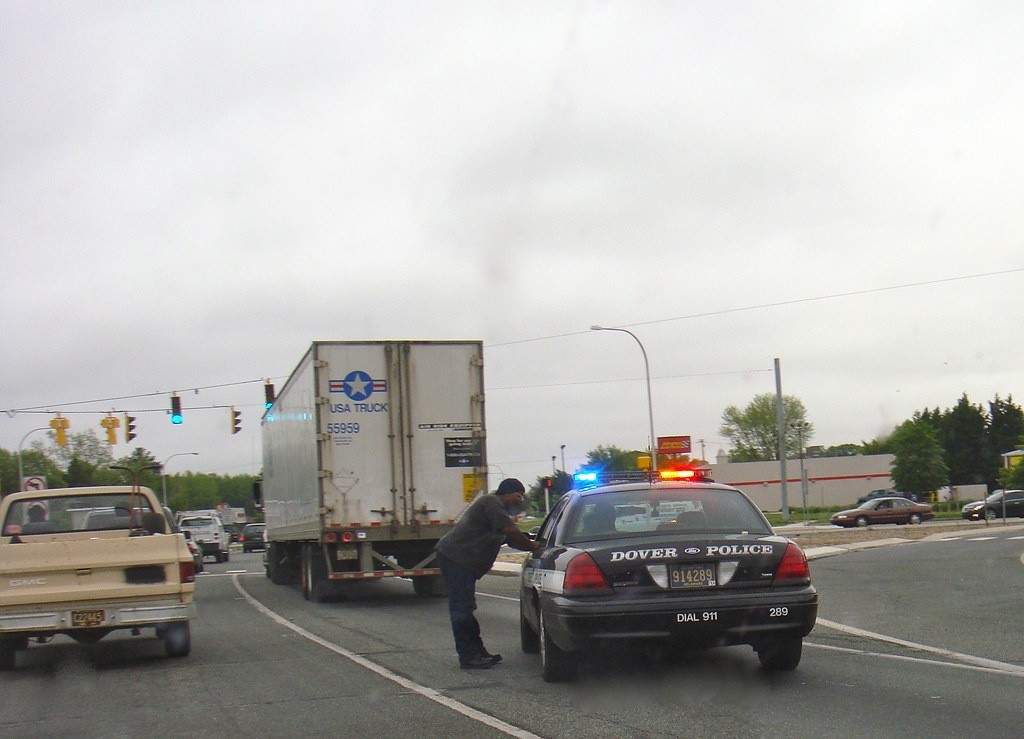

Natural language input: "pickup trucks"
[857,488,918,504]
[0,485,195,670]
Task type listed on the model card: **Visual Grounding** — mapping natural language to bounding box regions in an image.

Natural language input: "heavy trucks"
[253,340,492,605]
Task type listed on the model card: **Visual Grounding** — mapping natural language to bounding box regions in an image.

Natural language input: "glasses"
[514,491,525,502]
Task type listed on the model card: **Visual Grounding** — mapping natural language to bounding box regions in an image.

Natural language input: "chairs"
[110,516,137,529]
[22,520,54,534]
[677,510,707,530]
[582,513,616,533]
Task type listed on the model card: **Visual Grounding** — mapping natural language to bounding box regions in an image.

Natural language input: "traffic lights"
[545,478,552,488]
[265,384,275,408]
[230,410,241,435]
[172,397,183,425]
[125,416,136,443]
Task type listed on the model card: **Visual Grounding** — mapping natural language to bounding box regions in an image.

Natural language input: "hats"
[495,478,526,495]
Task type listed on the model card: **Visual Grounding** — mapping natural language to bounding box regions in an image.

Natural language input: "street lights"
[590,324,657,471]
[790,421,810,512]
[560,445,566,471]
[161,452,198,507]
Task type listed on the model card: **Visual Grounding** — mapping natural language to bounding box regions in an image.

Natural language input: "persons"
[115,501,136,518]
[596,501,617,528]
[27,505,48,522]
[434,477,546,670]
[911,493,918,503]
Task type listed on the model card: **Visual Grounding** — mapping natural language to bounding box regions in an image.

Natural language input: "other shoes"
[459,655,494,669]
[480,650,504,663]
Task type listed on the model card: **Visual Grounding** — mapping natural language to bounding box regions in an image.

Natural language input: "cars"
[239,523,267,553]
[79,505,232,574]
[829,497,936,527]
[962,490,1024,521]
[518,467,820,682]
